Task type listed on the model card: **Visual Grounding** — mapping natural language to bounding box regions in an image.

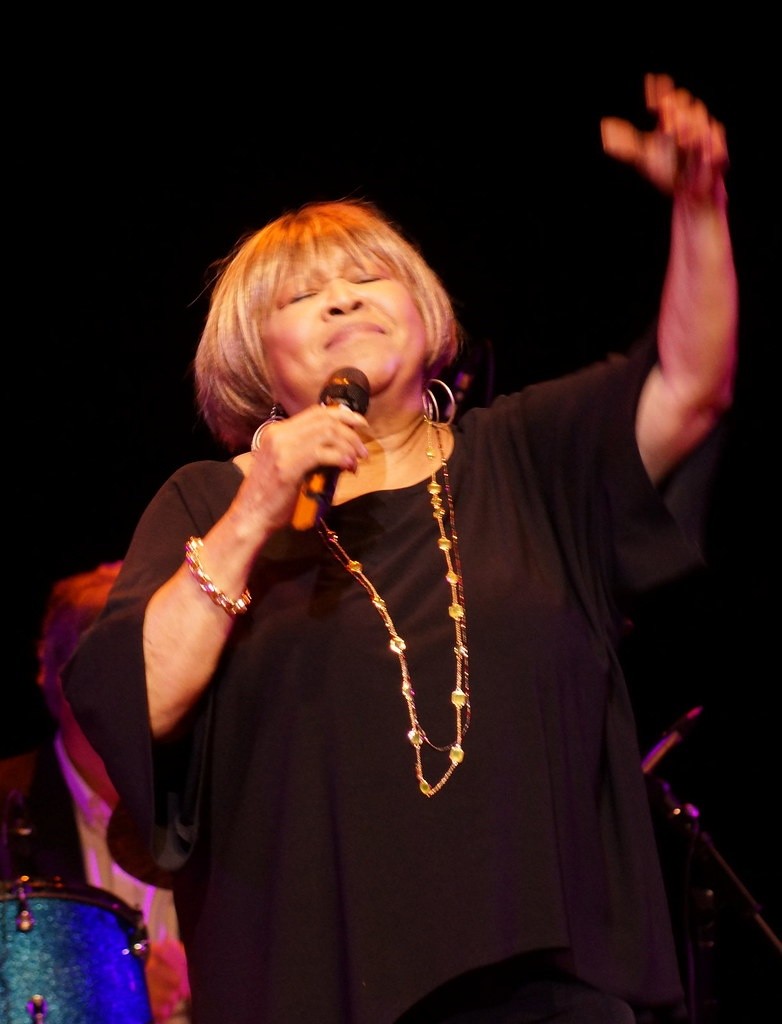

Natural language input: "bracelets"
[184,537,252,615]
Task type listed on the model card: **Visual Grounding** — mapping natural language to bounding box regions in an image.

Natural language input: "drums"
[0,880,157,1023]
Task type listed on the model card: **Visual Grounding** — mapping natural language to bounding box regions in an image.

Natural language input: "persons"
[62,77,738,1024]
[0,563,198,1024]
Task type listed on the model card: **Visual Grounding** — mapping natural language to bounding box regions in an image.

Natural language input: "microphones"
[293,366,371,532]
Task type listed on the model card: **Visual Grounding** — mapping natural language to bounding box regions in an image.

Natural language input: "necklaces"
[275,413,469,799]
